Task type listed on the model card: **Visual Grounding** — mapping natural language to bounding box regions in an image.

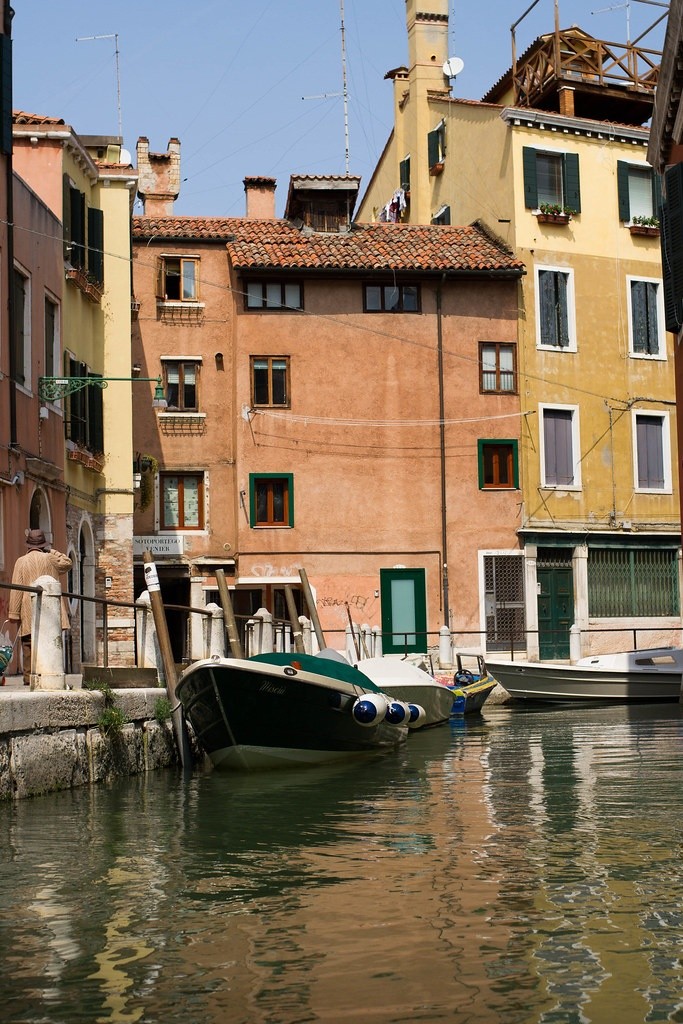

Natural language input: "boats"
[173,652,426,773]
[429,670,499,718]
[350,656,456,735]
[481,648,683,707]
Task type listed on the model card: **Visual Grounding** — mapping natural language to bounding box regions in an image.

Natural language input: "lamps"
[38,374,167,418]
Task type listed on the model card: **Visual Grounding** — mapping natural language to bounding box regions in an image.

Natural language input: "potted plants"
[84,450,111,474]
[630,216,660,237]
[133,454,158,513]
[65,263,88,291]
[81,277,104,304]
[68,440,89,465]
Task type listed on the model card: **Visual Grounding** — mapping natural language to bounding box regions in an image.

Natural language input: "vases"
[537,214,570,225]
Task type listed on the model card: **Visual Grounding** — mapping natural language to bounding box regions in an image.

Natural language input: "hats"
[26,530,48,548]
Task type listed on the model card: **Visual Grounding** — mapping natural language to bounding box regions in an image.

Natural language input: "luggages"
[0,619,21,685]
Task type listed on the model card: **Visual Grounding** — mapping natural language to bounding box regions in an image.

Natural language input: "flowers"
[541,201,572,215]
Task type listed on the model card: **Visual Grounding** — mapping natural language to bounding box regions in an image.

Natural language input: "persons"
[8,530,73,685]
